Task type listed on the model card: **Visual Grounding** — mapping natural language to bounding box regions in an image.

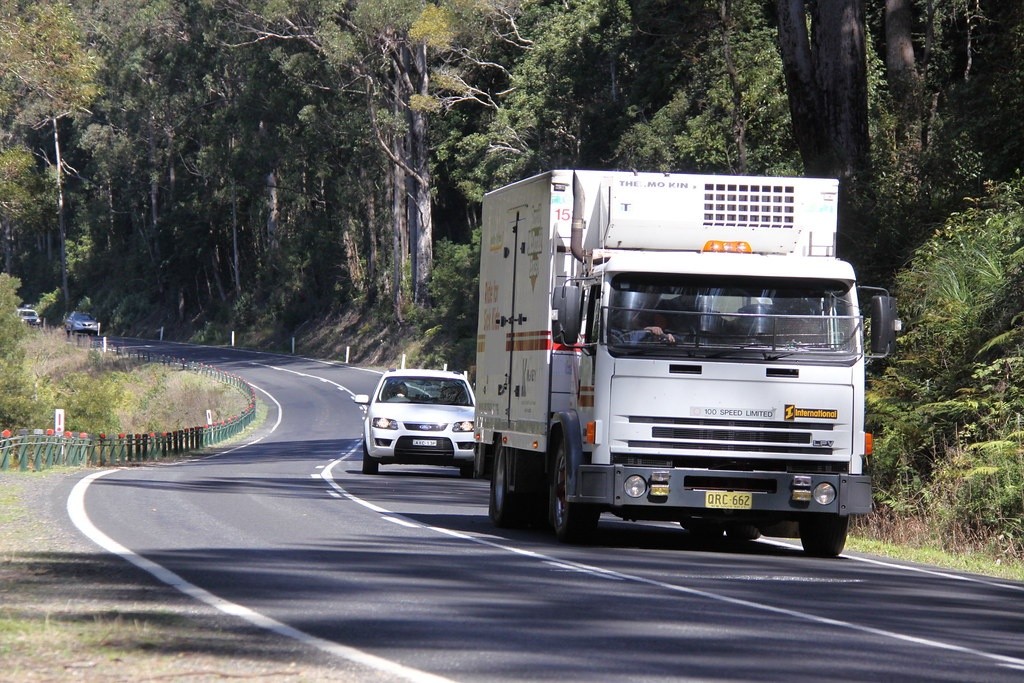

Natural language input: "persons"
[391,382,408,399]
[627,279,675,344]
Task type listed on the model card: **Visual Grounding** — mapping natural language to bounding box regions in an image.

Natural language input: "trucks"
[473,170,902,558]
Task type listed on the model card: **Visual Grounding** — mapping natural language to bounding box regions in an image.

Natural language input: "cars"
[64,310,99,336]
[354,367,476,479]
[15,308,42,325]
